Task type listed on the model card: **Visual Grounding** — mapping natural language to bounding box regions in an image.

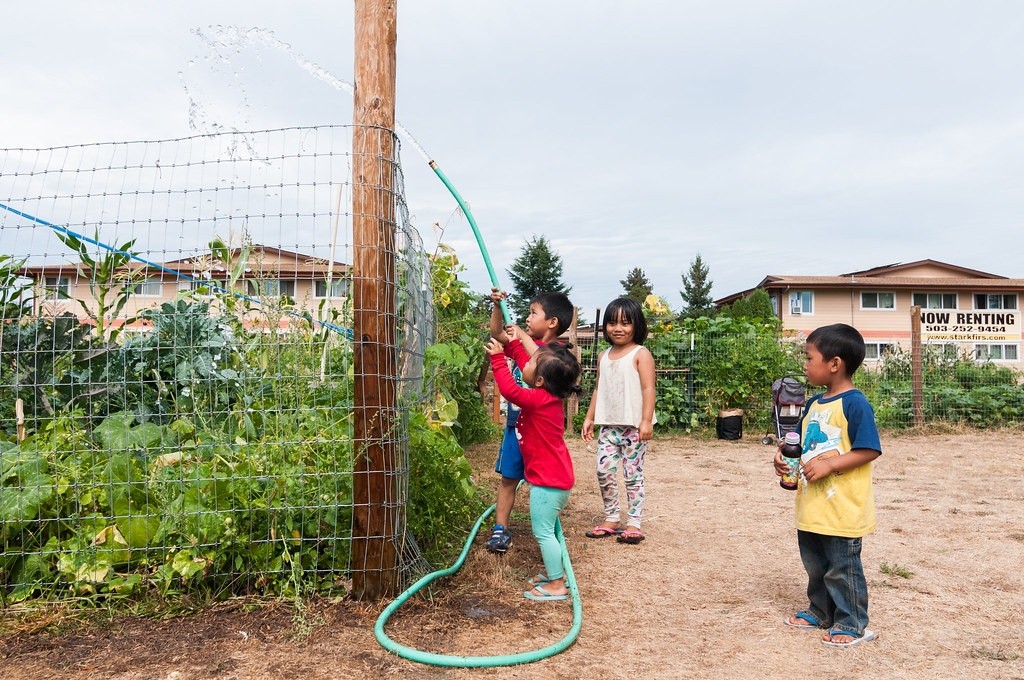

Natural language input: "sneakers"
[486,525,512,552]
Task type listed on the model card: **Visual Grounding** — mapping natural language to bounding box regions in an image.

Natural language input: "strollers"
[762,373,808,444]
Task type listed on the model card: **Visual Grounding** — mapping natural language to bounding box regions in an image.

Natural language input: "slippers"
[822,624,874,648]
[528,576,569,588]
[784,612,820,628]
[618,530,645,544]
[585,523,620,538]
[524,582,569,600]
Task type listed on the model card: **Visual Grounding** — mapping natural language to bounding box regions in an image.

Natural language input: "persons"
[580,298,656,544]
[484,328,580,600]
[774,323,882,647]
[486,287,574,554]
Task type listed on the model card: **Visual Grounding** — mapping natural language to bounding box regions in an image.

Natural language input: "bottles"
[780,432,802,490]
